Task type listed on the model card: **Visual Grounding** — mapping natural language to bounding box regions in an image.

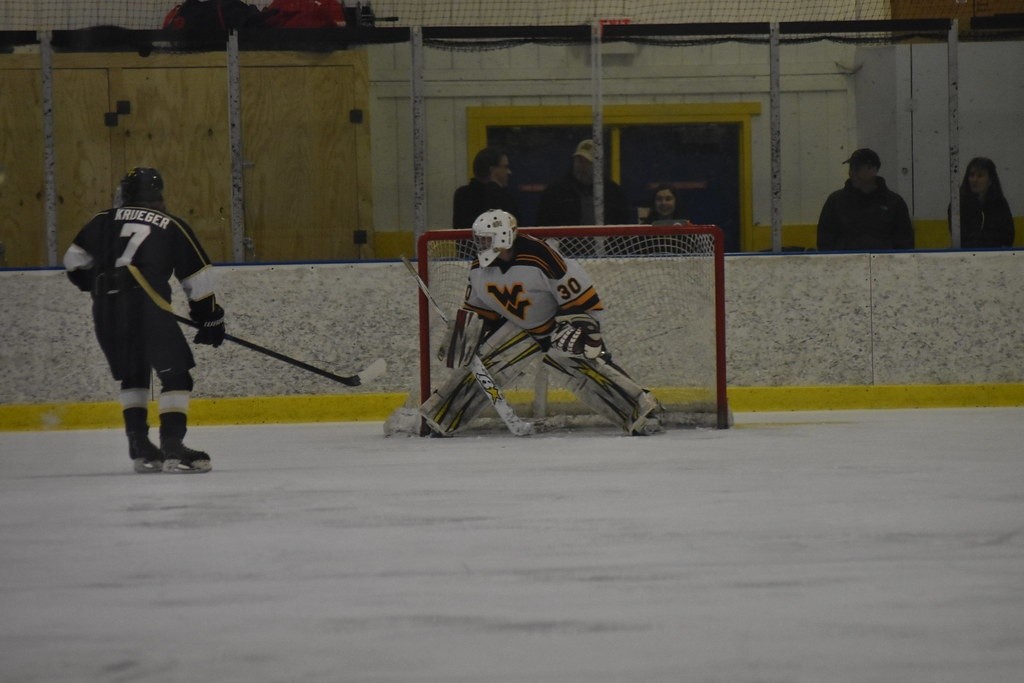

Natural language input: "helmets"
[472,209,517,266]
[120,167,162,201]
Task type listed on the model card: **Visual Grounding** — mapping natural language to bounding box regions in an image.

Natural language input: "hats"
[843,148,881,168]
[572,139,592,162]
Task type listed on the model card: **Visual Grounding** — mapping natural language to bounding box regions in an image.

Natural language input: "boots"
[126,431,212,474]
[630,404,667,436]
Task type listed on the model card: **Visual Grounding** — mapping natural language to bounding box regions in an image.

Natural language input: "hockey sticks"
[164,309,389,388]
[398,251,536,438]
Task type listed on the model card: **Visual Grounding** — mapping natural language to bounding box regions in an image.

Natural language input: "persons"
[612,163,665,201]
[535,139,629,257]
[453,140,542,254]
[64,162,226,472]
[947,157,1014,249]
[624,184,705,255]
[815,149,915,253]
[387,210,670,441]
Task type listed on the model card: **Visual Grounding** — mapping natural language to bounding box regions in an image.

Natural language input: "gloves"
[187,294,226,348]
